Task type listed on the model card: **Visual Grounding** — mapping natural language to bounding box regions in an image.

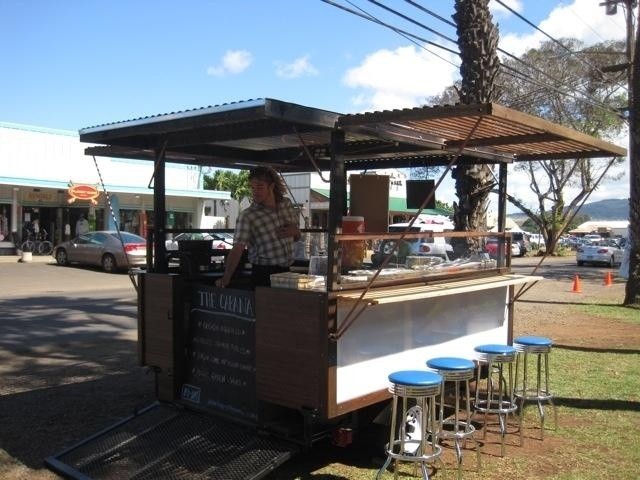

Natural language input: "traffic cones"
[603,270,613,286]
[572,274,582,293]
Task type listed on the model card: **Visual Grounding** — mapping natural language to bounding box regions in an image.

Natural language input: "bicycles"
[20,229,54,255]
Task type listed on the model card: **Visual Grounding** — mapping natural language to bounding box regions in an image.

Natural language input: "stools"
[376,370,447,480]
[470,344,524,458]
[425,356,482,480]
[514,336,558,441]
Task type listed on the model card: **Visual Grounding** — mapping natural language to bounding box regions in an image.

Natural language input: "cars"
[488,231,546,258]
[558,234,624,267]
[165,232,234,261]
[53,231,147,273]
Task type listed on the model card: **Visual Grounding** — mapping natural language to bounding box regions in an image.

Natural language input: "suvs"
[371,222,453,267]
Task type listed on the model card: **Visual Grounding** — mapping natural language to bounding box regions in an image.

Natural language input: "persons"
[214,165,302,291]
[75,213,89,236]
